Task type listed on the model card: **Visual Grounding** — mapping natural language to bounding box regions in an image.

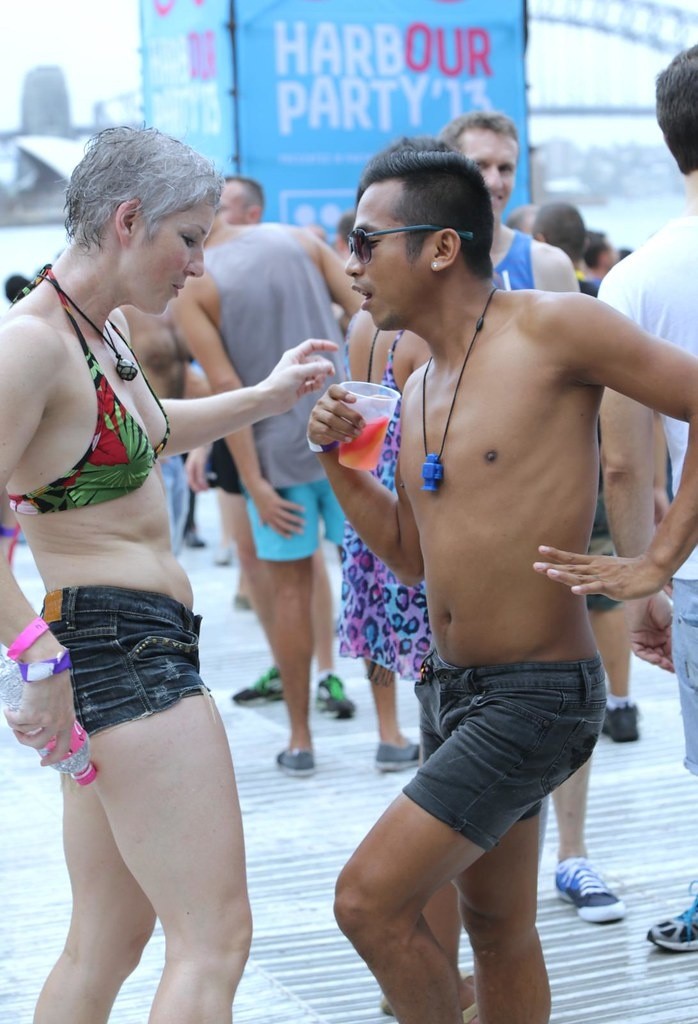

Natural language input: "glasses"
[347,224,474,264]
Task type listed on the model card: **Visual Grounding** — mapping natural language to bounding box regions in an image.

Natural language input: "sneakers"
[648,881,698,951]
[233,665,283,706]
[556,857,626,922]
[314,674,356,720]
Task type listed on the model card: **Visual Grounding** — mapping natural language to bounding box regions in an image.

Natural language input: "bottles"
[0,653,97,787]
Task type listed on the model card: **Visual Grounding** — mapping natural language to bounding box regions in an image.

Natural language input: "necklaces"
[39,273,137,381]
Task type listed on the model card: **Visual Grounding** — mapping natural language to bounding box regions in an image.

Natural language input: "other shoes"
[277,748,315,776]
[376,742,420,770]
[234,596,251,612]
[602,705,638,743]
[214,545,234,564]
[184,531,205,549]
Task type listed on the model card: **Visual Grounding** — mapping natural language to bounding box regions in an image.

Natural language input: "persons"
[506,203,634,743]
[307,153,698,1024]
[339,304,432,678]
[441,112,624,922]
[121,295,354,721]
[596,45,698,949]
[165,211,421,776]
[1,118,339,1024]
[336,214,354,258]
[6,274,31,302]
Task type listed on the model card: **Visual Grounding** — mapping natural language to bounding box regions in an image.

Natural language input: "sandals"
[382,973,477,1024]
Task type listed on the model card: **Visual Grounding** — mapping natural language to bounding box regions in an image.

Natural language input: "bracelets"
[6,618,50,661]
[308,439,337,452]
[18,649,72,683]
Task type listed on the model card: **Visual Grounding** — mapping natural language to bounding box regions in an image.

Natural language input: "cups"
[334,382,400,472]
